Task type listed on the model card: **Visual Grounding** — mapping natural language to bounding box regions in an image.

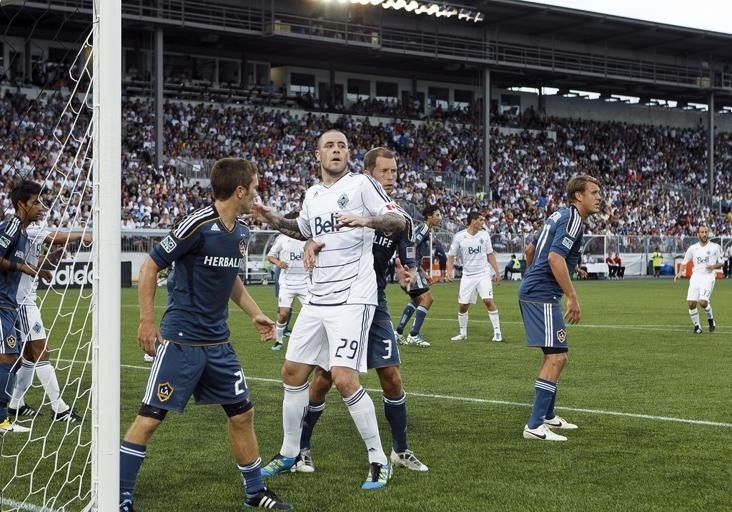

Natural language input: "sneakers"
[522,421,570,444]
[0,417,33,439]
[244,485,293,511]
[270,341,284,351]
[362,461,395,492]
[491,334,503,342]
[450,334,469,342]
[119,494,137,512]
[390,448,430,476]
[142,353,155,363]
[8,403,45,420]
[406,333,431,349]
[283,328,293,338]
[544,414,579,432]
[51,408,87,426]
[708,320,716,333]
[393,331,409,348]
[693,325,702,336]
[261,452,297,480]
[294,448,316,476]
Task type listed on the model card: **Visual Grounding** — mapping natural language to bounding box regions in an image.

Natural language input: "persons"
[444,212,503,343]
[392,172,483,228]
[615,253,626,280]
[243,131,417,491]
[266,212,313,350]
[295,148,429,473]
[264,215,296,336]
[393,206,443,349]
[395,91,485,171]
[239,186,310,230]
[366,94,395,149]
[316,87,347,135]
[0,172,97,251]
[432,238,448,283]
[0,55,214,175]
[214,70,255,158]
[651,247,663,280]
[482,180,569,254]
[519,174,604,442]
[582,213,598,236]
[599,120,658,250]
[584,252,595,263]
[255,80,316,134]
[255,134,320,185]
[503,255,520,280]
[708,121,732,244]
[673,227,724,335]
[454,254,464,277]
[347,91,366,175]
[489,105,599,179]
[8,192,93,424]
[119,156,294,512]
[0,179,52,433]
[659,125,707,251]
[386,249,398,284]
[606,251,618,280]
[117,175,215,251]
[721,248,729,279]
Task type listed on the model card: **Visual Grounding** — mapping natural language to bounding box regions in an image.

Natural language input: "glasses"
[122,214,130,218]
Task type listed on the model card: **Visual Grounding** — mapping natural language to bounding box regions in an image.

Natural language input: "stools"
[511,273,521,281]
